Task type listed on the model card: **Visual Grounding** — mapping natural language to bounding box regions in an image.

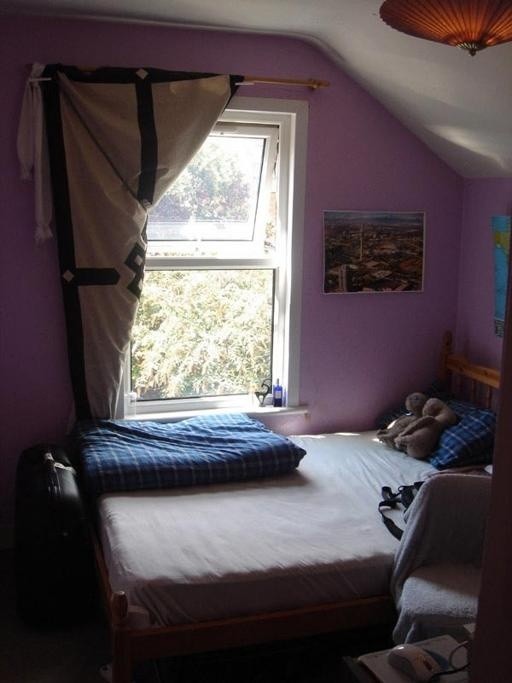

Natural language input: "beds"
[79,330,500,683]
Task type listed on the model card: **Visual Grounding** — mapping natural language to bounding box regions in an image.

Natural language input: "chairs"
[391,474,492,645]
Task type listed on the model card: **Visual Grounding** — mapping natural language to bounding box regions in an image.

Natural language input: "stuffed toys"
[395,397,456,458]
[376,392,426,450]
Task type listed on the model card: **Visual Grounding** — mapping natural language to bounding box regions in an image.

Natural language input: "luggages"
[16,441,87,631]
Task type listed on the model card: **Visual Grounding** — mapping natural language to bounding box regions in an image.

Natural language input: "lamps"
[379,1,511,55]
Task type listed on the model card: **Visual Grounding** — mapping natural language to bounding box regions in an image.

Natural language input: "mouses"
[388,643,449,683]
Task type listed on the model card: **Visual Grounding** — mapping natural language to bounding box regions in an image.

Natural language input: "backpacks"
[378,481,423,541]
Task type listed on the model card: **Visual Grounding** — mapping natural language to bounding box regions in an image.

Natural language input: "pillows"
[380,388,496,470]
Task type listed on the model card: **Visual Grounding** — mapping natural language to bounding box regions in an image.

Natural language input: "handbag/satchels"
[377,466,491,544]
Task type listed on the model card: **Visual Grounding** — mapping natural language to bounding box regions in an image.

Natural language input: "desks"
[340,623,474,683]
